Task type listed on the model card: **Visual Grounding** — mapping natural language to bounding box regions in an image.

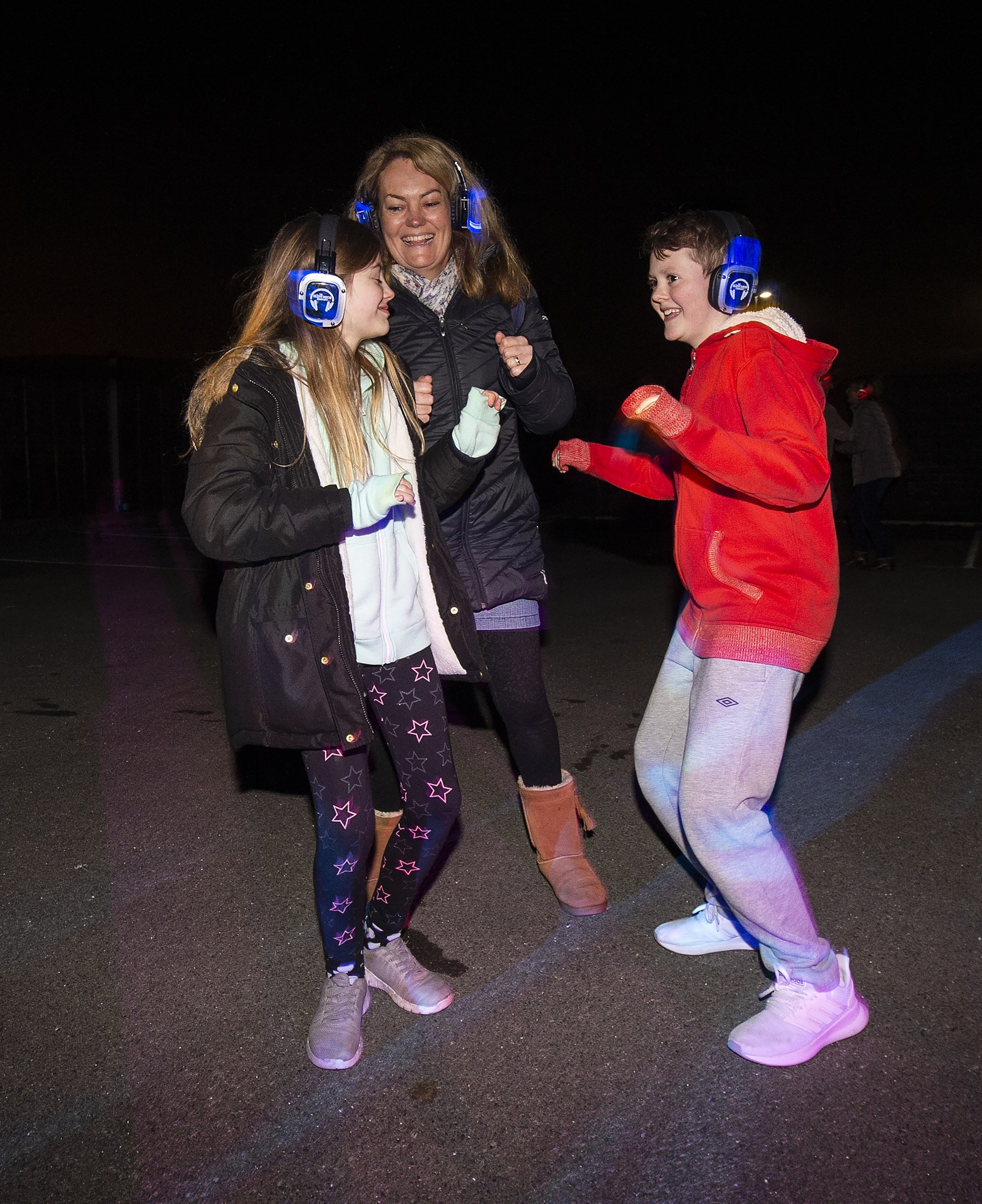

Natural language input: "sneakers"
[304,964,371,1069]
[727,947,869,1066]
[364,933,455,1014]
[653,903,758,955]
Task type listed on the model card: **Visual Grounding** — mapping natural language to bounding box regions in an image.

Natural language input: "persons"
[345,135,609,915]
[551,211,868,1067]
[180,214,504,1072]
[819,372,903,573]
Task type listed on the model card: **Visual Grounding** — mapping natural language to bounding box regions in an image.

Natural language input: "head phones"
[286,214,346,327]
[708,211,762,315]
[354,161,486,235]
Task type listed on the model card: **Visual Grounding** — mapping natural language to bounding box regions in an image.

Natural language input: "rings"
[514,356,520,366]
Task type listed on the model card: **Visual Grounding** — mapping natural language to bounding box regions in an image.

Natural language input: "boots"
[516,769,608,915]
[367,809,404,902]
